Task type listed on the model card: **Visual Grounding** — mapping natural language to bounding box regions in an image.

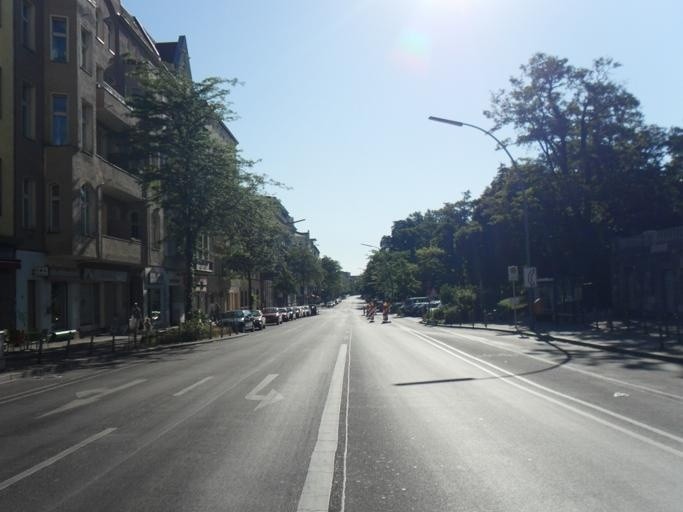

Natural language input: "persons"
[131,302,143,333]
[207,300,216,322]
[144,318,152,332]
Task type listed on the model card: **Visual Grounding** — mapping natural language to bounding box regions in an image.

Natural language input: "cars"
[216,297,342,334]
[370,297,439,318]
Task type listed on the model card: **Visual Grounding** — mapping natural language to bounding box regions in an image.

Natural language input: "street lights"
[360,242,381,251]
[427,115,536,329]
